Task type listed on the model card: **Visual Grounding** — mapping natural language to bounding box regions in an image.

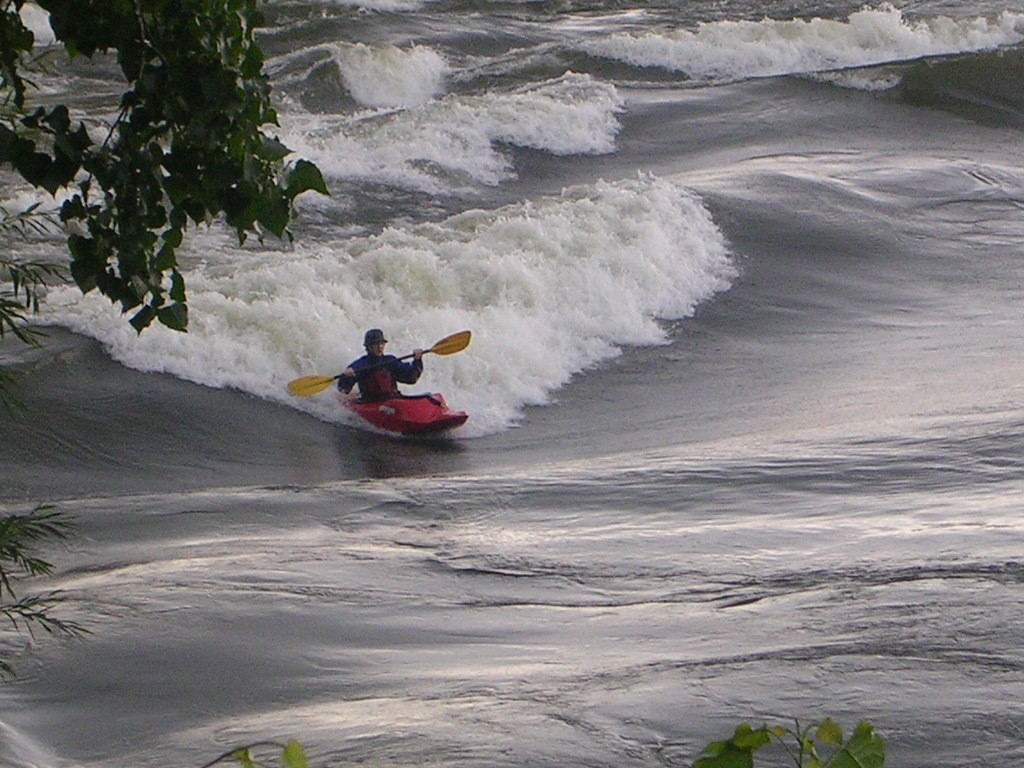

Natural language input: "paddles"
[287,329,472,397]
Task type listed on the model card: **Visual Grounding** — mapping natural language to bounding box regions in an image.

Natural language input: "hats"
[363,329,388,346]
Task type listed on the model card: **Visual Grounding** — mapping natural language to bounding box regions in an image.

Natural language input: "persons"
[337,329,423,400]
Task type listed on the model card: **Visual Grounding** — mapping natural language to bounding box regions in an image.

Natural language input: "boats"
[333,383,470,437]
